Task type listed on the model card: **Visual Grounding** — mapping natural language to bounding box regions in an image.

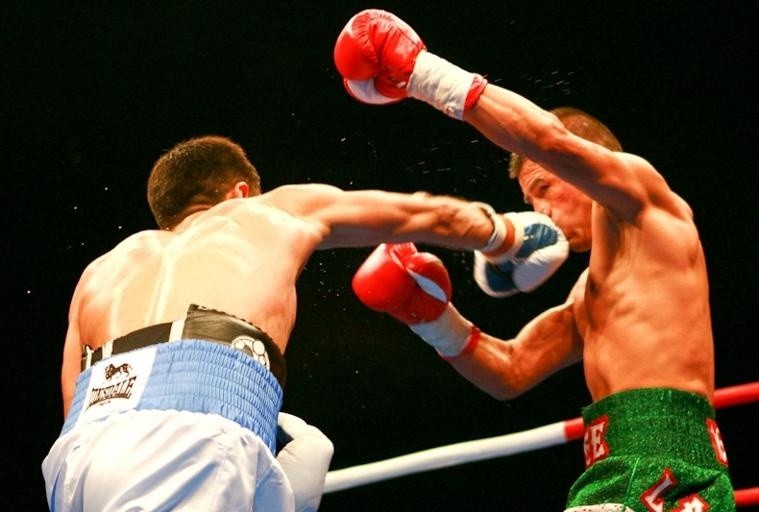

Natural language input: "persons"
[40,133,572,511]
[333,10,737,510]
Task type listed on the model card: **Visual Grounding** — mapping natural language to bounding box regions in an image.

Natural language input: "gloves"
[473,199,571,297]
[333,8,487,120]
[274,410,335,512]
[352,241,480,361]
